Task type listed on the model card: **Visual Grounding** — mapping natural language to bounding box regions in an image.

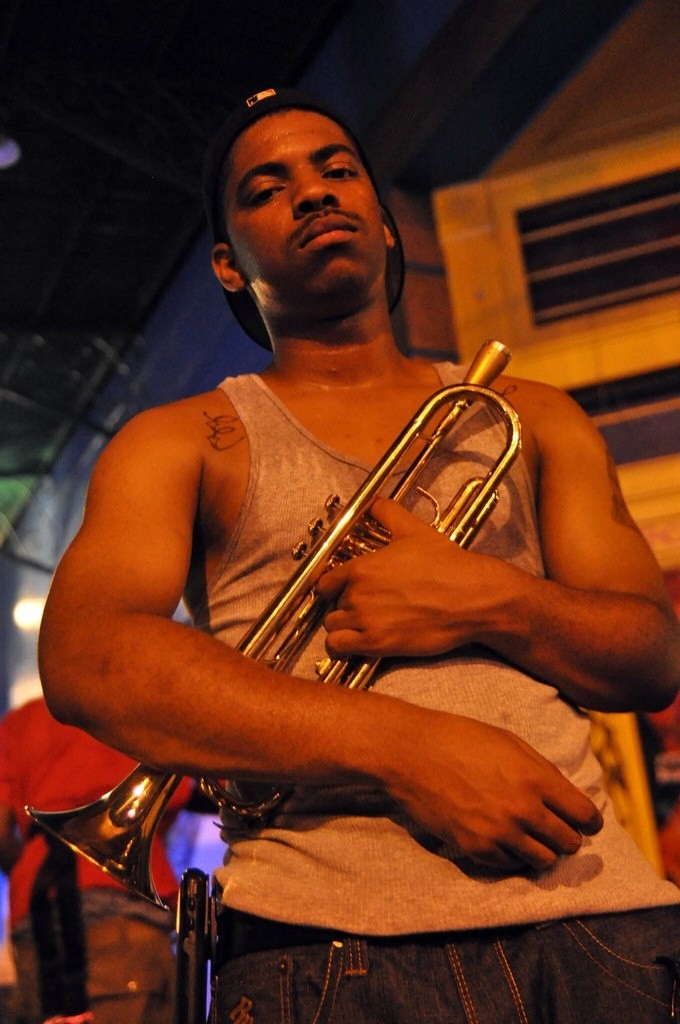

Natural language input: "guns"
[174,865,209,1024]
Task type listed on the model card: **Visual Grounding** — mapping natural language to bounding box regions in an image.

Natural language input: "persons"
[0,697,230,1024]
[38,88,680,1024]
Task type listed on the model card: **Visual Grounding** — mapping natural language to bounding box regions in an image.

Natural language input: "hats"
[201,89,404,354]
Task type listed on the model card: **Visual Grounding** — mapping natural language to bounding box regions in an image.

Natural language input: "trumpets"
[19,334,528,916]
